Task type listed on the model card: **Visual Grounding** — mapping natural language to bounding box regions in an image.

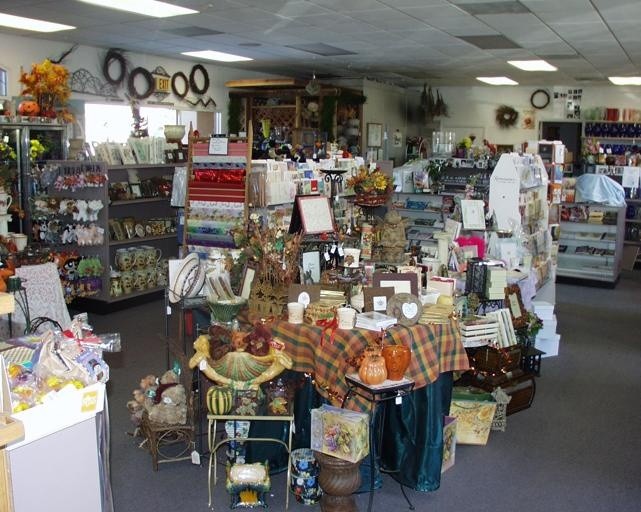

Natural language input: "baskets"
[478,347,522,369]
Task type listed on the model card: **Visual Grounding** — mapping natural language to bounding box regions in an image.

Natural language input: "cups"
[336,308,355,329]
[287,302,305,325]
[0,192,13,216]
[108,246,169,296]
[343,247,361,268]
[15,235,28,251]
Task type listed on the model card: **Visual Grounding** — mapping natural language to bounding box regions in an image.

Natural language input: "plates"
[168,252,206,304]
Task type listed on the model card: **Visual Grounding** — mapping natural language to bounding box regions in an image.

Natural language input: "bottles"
[188,121,194,162]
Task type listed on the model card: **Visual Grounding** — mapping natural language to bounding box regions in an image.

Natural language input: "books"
[459,307,519,349]
[355,312,399,332]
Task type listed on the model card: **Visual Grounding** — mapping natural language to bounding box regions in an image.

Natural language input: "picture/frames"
[288,284,320,318]
[296,196,335,235]
[363,273,423,326]
[367,123,383,148]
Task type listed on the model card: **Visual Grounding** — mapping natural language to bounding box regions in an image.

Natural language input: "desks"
[235,310,469,493]
[339,373,415,512]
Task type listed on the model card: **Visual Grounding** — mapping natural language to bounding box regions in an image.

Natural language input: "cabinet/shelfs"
[1,327,114,512]
[391,139,561,360]
[538,121,641,290]
[207,415,294,512]
[163,287,237,469]
[0,123,70,251]
[46,161,188,315]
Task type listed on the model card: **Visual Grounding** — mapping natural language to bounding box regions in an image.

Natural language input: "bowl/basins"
[164,124,185,140]
[205,298,244,324]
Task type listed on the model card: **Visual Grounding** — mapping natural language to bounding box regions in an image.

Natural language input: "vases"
[314,451,363,512]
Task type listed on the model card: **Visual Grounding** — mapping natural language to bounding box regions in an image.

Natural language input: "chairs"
[124,336,204,472]
